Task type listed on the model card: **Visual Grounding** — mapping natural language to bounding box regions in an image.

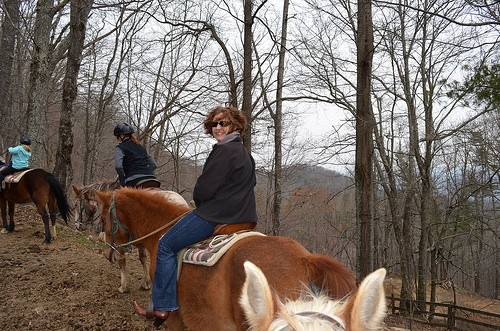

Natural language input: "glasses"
[210,120,231,127]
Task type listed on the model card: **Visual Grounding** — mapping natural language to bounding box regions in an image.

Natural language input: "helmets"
[20,136,32,145]
[113,122,135,136]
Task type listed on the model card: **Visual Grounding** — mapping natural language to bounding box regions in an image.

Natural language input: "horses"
[71,183,191,293]
[0,155,72,243]
[94,188,387,331]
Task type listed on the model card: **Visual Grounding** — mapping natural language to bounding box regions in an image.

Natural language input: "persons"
[133,106,258,330]
[0,137,33,184]
[97,122,158,243]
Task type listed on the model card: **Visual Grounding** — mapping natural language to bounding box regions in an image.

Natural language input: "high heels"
[132,299,170,328]
